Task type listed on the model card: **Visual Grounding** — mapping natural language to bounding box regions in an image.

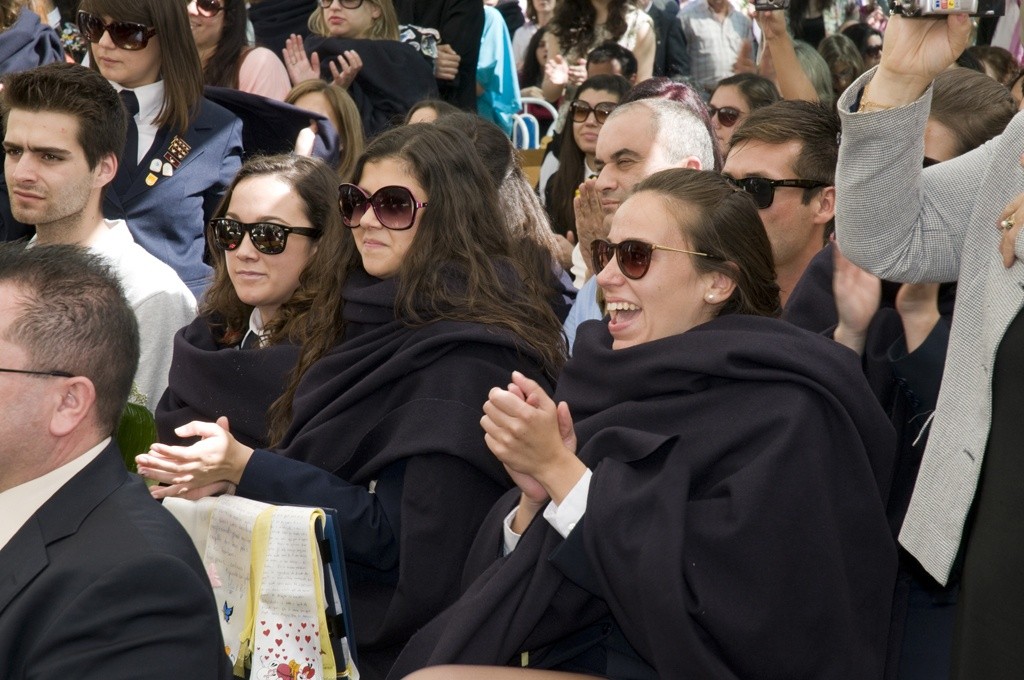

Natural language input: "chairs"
[153,490,360,680]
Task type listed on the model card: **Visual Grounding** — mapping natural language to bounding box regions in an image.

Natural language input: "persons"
[132,121,569,679]
[152,152,357,458]
[0,0,1022,358]
[0,64,201,418]
[724,98,842,319]
[833,0,1024,680]
[781,65,1018,536]
[0,240,243,680]
[380,168,899,680]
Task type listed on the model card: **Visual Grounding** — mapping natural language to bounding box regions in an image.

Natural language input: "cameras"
[754,0,791,11]
[887,0,1005,19]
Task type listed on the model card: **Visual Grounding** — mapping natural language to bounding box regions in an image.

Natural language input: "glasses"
[0,4,19,30]
[720,176,829,209]
[705,105,746,128]
[75,10,156,50]
[337,184,427,230]
[591,239,716,279]
[186,1,224,19]
[568,100,615,123]
[209,218,322,255]
[315,1,365,9]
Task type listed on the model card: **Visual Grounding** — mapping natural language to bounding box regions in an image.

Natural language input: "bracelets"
[856,82,894,115]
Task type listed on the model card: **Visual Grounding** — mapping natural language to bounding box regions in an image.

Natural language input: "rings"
[1000,213,1015,230]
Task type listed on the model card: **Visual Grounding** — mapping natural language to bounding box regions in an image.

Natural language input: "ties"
[117,90,140,173]
